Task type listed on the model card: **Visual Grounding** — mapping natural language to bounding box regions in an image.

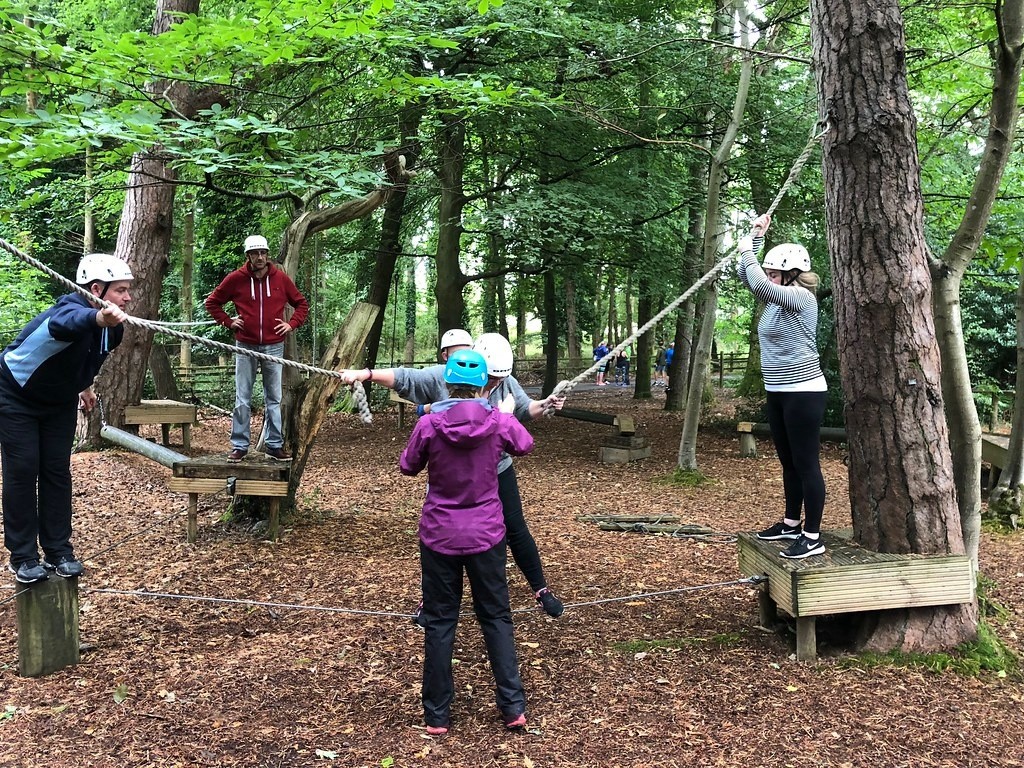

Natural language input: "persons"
[736,215,829,558]
[653,339,676,390]
[440,328,472,362]
[592,341,629,386]
[398,350,536,733]
[0,255,135,582]
[205,235,307,460]
[340,330,565,623]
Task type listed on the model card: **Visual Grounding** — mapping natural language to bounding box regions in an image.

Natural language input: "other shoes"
[622,384,631,388]
[658,382,665,386]
[424,716,448,734]
[621,381,624,385]
[652,381,658,386]
[615,383,618,387]
[596,381,599,385]
[502,704,526,726]
[598,381,606,385]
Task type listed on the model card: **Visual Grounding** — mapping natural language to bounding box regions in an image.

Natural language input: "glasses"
[248,251,268,257]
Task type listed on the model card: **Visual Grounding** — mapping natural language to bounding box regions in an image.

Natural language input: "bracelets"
[364,367,372,380]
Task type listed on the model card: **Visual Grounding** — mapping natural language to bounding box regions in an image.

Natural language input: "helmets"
[244,235,270,253]
[440,329,472,350]
[472,332,513,377]
[761,243,811,272]
[76,253,135,285]
[444,349,489,386]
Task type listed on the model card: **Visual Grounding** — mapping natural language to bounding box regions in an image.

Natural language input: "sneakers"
[536,588,565,617]
[779,532,825,559]
[43,553,85,577]
[8,560,49,583]
[756,518,804,540]
[415,598,426,629]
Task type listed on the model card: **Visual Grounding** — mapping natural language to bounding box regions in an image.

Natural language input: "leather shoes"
[265,444,293,461]
[226,449,248,462]
